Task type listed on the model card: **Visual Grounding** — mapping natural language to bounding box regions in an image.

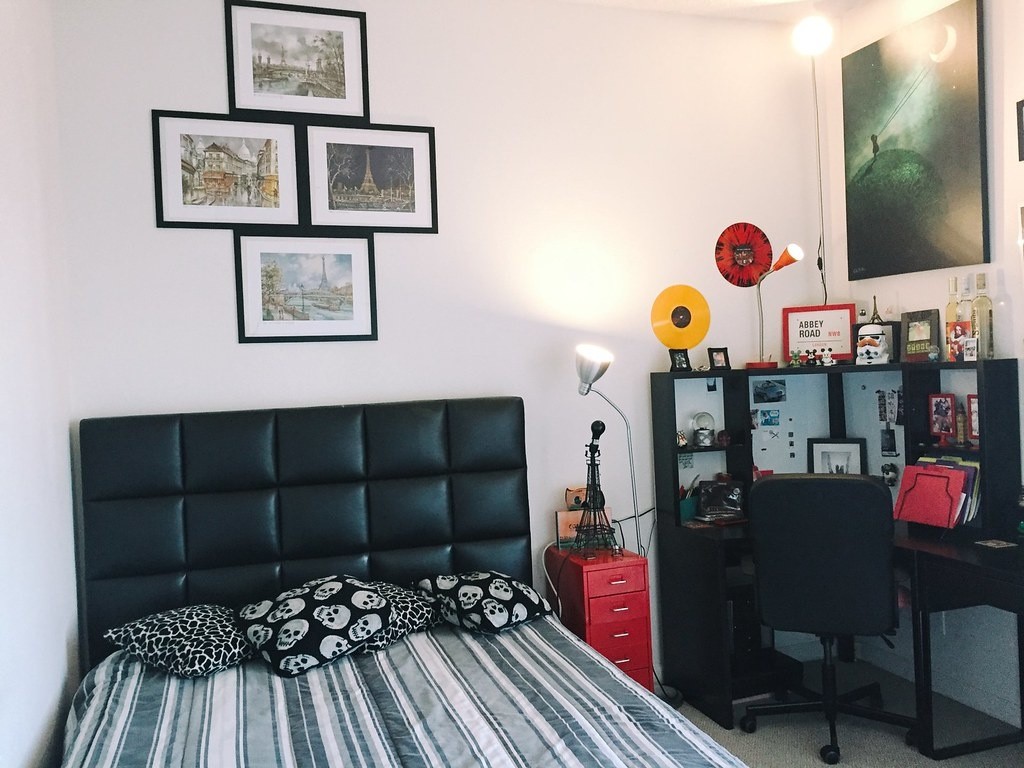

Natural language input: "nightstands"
[545,545,655,694]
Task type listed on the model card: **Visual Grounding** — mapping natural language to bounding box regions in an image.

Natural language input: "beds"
[60,395,749,768]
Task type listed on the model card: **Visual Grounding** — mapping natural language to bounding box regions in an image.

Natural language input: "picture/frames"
[900,308,940,361]
[967,394,979,439]
[669,348,692,372]
[223,0,371,124]
[302,123,439,234]
[232,230,379,344]
[806,438,870,476]
[151,109,301,230]
[708,347,732,370]
[782,303,855,362]
[929,393,957,437]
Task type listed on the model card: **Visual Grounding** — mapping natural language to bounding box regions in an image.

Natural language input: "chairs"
[739,472,919,765]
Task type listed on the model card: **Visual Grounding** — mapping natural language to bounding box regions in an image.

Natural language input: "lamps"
[574,343,644,556]
[745,242,804,370]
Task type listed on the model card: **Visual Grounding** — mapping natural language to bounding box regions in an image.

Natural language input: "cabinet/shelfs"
[649,357,1023,536]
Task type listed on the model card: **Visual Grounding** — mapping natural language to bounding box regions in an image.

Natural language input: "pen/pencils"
[680,473,700,499]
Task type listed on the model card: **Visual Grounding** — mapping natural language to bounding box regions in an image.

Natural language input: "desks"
[656,517,1024,761]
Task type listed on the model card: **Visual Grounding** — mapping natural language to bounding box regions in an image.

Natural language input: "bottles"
[945,276,960,362]
[970,272,995,361]
[956,274,972,362]
[956,402,968,445]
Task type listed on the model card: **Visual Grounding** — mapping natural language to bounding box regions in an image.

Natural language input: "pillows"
[103,603,255,680]
[234,573,398,679]
[357,579,447,656]
[408,569,553,636]
